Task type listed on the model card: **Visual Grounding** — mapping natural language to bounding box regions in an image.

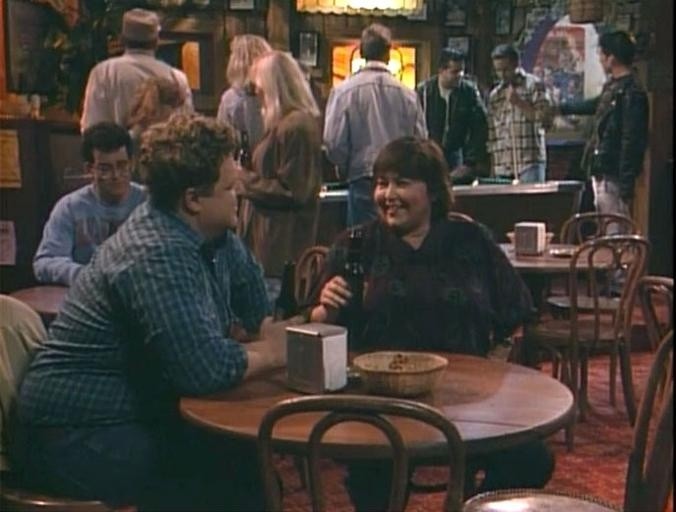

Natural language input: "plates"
[342,365,364,384]
[547,248,577,258]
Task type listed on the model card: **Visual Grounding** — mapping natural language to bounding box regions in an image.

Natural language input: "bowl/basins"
[505,230,554,250]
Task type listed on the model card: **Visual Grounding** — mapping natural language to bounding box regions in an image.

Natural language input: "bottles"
[336,225,367,327]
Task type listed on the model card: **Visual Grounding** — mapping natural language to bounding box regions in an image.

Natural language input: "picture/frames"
[297,31,319,68]
[228,0,255,12]
[443,0,513,80]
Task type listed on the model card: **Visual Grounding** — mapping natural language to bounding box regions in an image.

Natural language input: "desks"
[317,180,586,247]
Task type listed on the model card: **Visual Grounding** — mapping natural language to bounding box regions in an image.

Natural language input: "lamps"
[297,0,423,17]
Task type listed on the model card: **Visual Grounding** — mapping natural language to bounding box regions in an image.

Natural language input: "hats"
[123,8,161,43]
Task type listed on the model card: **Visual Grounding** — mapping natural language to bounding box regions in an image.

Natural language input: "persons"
[418,46,487,184]
[551,29,650,297]
[325,23,428,228]
[303,136,555,511]
[486,44,550,186]
[233,49,321,318]
[79,8,194,185]
[215,34,274,168]
[11,109,307,511]
[33,121,151,288]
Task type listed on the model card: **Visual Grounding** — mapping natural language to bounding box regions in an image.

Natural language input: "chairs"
[292,246,331,308]
[520,211,652,428]
[465,328,674,511]
[0,293,135,512]
[636,275,675,408]
[260,393,465,512]
